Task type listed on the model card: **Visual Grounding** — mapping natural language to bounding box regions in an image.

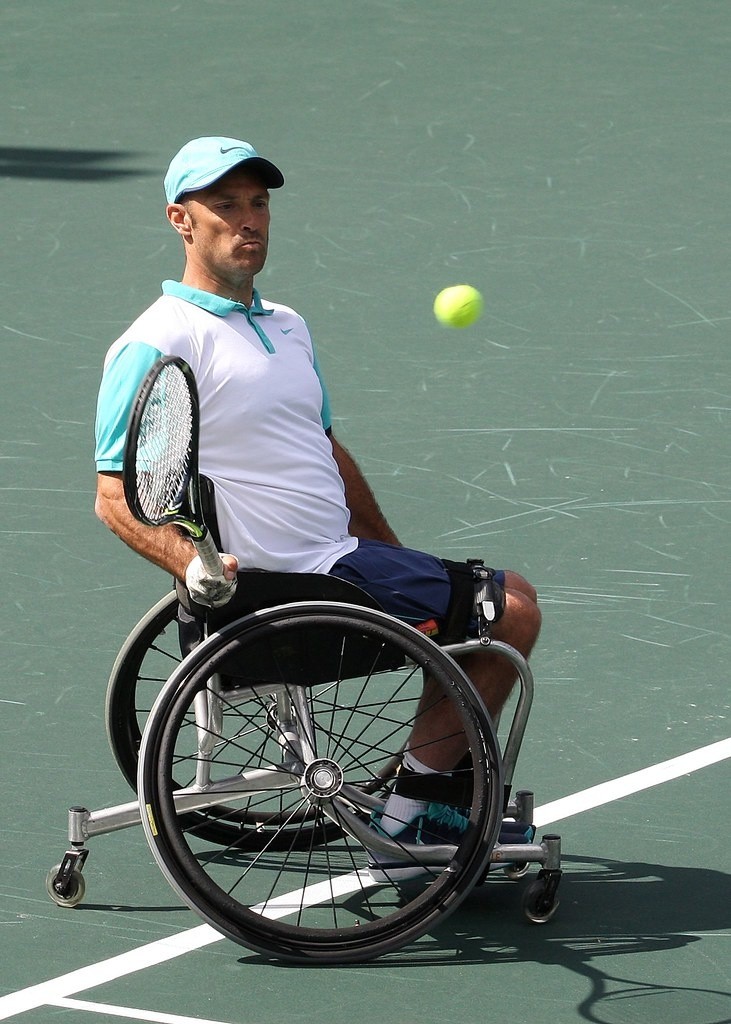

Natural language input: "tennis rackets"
[122,354,226,576]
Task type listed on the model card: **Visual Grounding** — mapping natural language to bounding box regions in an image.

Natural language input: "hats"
[164,137,285,204]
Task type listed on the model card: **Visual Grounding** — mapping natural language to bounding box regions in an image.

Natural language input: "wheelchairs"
[45,473,564,968]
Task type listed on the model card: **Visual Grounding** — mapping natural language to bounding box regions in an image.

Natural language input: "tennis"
[432,284,484,330]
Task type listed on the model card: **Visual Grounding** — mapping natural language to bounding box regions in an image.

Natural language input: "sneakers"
[367,802,528,883]
[370,806,534,846]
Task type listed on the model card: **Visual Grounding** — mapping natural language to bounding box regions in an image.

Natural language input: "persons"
[92,135,541,884]
